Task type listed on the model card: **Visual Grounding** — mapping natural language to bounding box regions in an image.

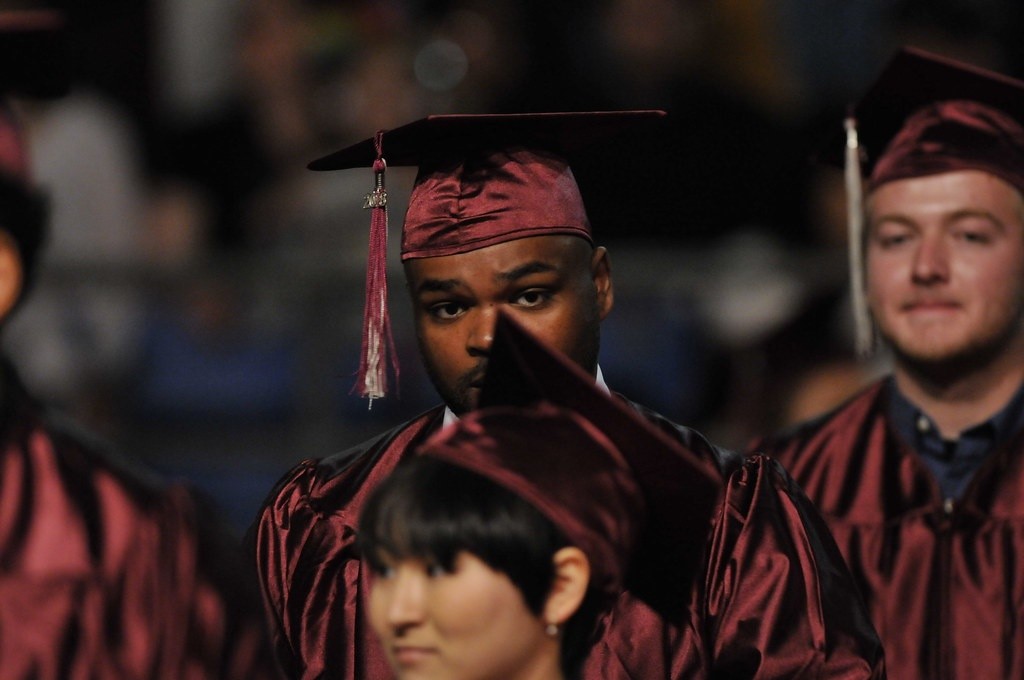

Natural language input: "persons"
[748,45,1024,680]
[0,117,275,680]
[356,306,726,680]
[247,110,888,680]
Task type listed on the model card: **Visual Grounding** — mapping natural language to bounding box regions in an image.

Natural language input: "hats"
[405,311,727,642]
[305,110,668,400]
[809,43,1024,349]
